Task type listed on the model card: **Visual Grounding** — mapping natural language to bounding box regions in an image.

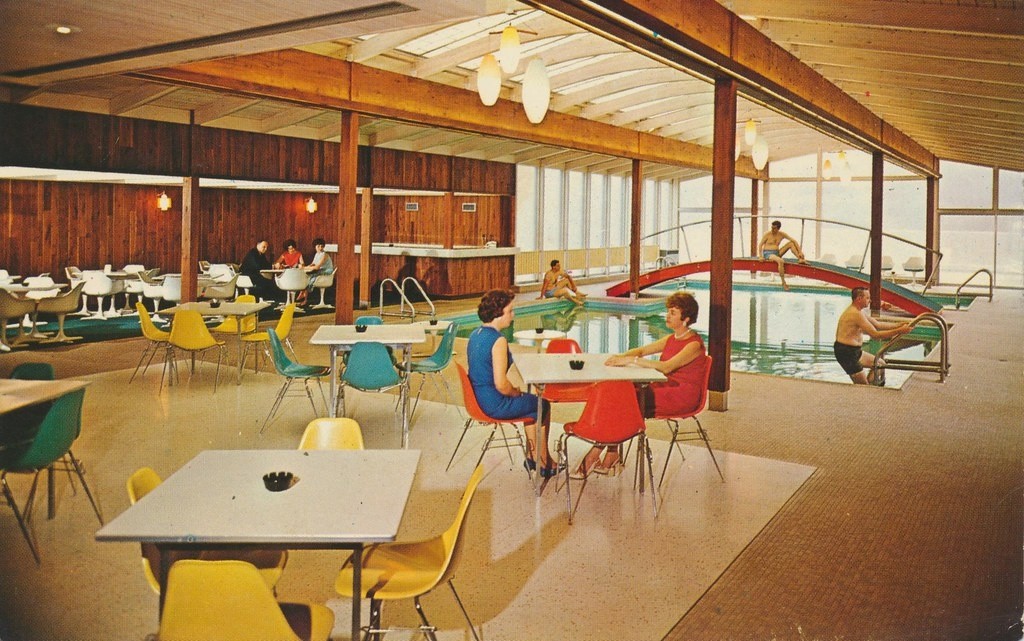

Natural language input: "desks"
[309,325,427,425]
[94,450,424,639]
[260,265,326,310]
[150,272,210,280]
[153,301,272,385]
[510,353,668,494]
[0,377,93,518]
[73,270,137,318]
[3,282,69,346]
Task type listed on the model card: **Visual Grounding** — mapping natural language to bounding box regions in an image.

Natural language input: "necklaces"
[673,329,691,339]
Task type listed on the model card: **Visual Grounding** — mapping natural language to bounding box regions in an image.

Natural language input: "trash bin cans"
[660,250,679,268]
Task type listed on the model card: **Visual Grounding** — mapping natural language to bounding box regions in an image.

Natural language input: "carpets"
[0,297,441,355]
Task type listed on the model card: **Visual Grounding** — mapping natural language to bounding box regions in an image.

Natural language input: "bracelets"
[634,355,638,363]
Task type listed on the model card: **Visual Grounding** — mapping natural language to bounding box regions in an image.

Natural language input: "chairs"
[815,254,837,265]
[901,257,926,289]
[845,254,867,274]
[882,256,895,284]
[0,260,727,640]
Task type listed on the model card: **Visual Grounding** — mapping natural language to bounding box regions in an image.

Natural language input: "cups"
[298,264,302,268]
[104,264,112,274]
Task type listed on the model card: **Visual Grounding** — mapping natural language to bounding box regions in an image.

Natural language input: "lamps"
[476,13,551,125]
[735,118,770,171]
[822,149,855,183]
[156,190,173,212]
[305,196,318,213]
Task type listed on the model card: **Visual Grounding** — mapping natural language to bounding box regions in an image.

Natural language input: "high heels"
[569,458,602,480]
[524,459,537,480]
[540,463,566,476]
[594,457,620,477]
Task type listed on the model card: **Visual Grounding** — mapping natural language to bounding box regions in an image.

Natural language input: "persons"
[274,240,304,269]
[569,291,706,481]
[297,237,333,306]
[759,220,811,291]
[466,287,564,480]
[536,259,589,306]
[239,240,278,317]
[832,286,914,384]
